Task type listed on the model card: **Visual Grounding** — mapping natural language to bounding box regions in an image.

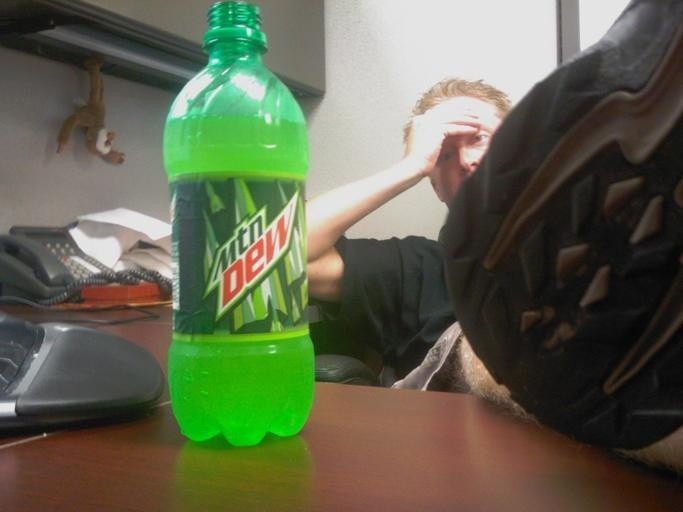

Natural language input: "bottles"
[161,1,318,448]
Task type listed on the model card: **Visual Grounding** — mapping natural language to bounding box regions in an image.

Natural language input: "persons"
[305,0,682,477]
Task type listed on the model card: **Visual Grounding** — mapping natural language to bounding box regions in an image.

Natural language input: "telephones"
[0,226,116,298]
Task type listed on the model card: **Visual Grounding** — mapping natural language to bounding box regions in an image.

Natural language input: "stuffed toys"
[54,57,125,166]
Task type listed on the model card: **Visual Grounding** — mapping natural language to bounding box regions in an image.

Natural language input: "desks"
[0,301,681,509]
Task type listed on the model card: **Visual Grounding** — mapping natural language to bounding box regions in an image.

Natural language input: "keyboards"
[1,313,165,436]
